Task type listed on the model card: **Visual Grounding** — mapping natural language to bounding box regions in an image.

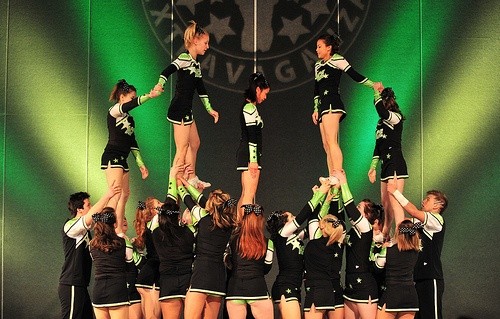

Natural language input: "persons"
[146,20,219,189]
[101,78,165,239]
[58,158,449,319]
[312,33,384,186]
[236,73,271,221]
[367,81,408,240]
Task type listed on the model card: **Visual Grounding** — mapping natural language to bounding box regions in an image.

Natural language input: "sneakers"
[188,177,211,188]
[319,177,339,185]
[117,233,128,240]
